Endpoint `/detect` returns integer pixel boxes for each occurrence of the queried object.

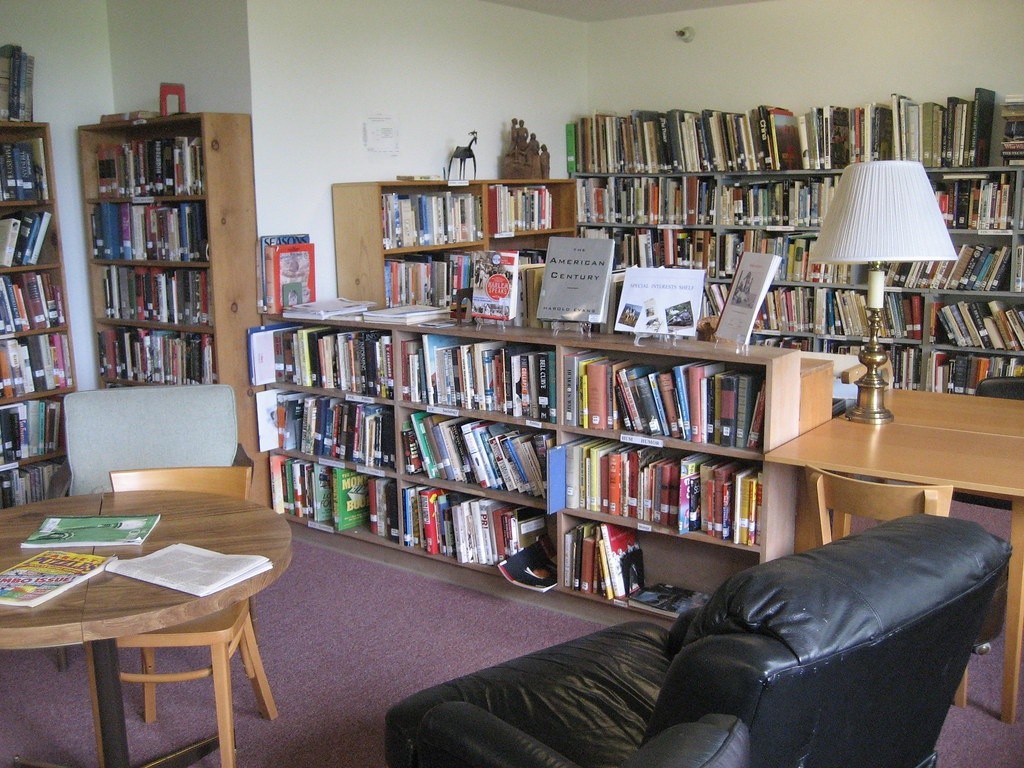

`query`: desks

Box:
[0,490,292,768]
[837,387,1024,437]
[765,417,1024,724]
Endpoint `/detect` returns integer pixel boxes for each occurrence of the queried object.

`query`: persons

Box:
[512,118,550,179]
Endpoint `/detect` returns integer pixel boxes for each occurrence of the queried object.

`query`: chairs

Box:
[952,377,1024,511]
[805,464,969,707]
[85,465,279,768]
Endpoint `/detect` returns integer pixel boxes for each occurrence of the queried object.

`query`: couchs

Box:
[385,515,1013,768]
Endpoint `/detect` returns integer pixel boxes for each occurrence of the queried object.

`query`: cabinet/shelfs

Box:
[0,122,77,473]
[332,179,577,307]
[77,113,271,509]
[799,358,834,435]
[571,166,1024,392]
[261,307,800,623]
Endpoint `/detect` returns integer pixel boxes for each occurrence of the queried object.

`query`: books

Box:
[0,210,52,268]
[563,87,1024,170]
[699,286,1024,400]
[0,550,119,607]
[579,225,1022,295]
[92,138,206,198]
[0,45,37,124]
[244,322,765,450]
[20,513,161,548]
[0,143,48,206]
[97,264,215,325]
[0,403,62,465]
[254,388,762,546]
[0,273,66,335]
[0,327,77,403]
[268,452,712,618]
[257,230,785,352]
[100,329,214,384]
[574,172,1012,234]
[92,202,210,263]
[0,461,69,509]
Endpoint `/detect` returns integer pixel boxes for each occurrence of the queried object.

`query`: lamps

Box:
[809,160,959,425]
